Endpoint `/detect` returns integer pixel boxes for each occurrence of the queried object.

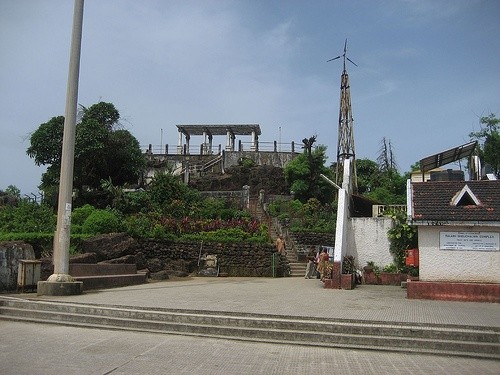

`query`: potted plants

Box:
[382,264,400,286]
[341,255,355,290]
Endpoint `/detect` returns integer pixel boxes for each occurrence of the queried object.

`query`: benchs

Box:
[69,263,146,291]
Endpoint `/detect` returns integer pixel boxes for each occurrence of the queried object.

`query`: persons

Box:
[276,234,286,254]
[304,246,329,279]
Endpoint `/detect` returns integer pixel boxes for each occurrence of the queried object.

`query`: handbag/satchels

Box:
[317,271,320,279]
